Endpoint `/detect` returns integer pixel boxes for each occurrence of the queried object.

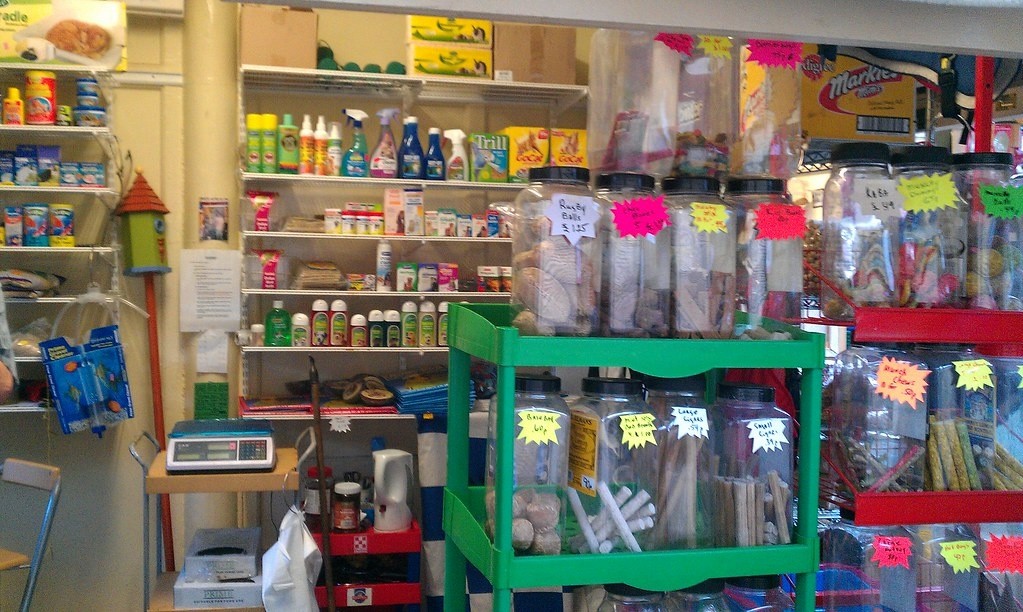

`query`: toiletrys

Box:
[250,299,448,347]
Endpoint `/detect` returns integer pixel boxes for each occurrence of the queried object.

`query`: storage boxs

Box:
[492,23,576,84]
[239,6,318,70]
[466,125,588,186]
[182,524,259,578]
[171,572,264,612]
[383,187,499,239]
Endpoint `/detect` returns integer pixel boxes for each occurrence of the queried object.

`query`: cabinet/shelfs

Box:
[803,307,1023,526]
[442,296,828,611]
[238,65,587,530]
[0,58,123,413]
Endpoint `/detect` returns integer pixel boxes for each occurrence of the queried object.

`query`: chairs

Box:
[0,459,62,612]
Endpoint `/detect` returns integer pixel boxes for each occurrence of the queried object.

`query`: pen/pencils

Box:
[363,478,371,490]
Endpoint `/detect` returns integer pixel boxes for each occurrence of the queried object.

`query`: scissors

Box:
[343,471,361,483]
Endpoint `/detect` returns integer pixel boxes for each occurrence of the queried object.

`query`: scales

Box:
[166,418,275,475]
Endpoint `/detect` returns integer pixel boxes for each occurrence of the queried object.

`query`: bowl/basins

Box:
[72,79,106,127]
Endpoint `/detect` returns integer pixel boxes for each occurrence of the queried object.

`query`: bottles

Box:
[247,113,277,174]
[251,324,264,347]
[265,301,291,347]
[587,28,802,177]
[818,342,1023,612]
[571,376,662,554]
[343,211,385,235]
[376,240,392,292]
[402,300,471,347]
[398,117,425,178]
[277,115,299,174]
[303,466,360,534]
[292,300,368,348]
[425,127,445,179]
[300,115,314,175]
[596,576,794,612]
[786,143,1023,315]
[643,373,792,549]
[485,373,572,556]
[315,115,330,175]
[511,167,805,343]
[3,70,56,125]
[328,123,343,176]
[368,310,402,347]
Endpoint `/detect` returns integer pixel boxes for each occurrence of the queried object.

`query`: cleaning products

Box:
[375,239,393,291]
[246,107,470,182]
[2,87,24,125]
